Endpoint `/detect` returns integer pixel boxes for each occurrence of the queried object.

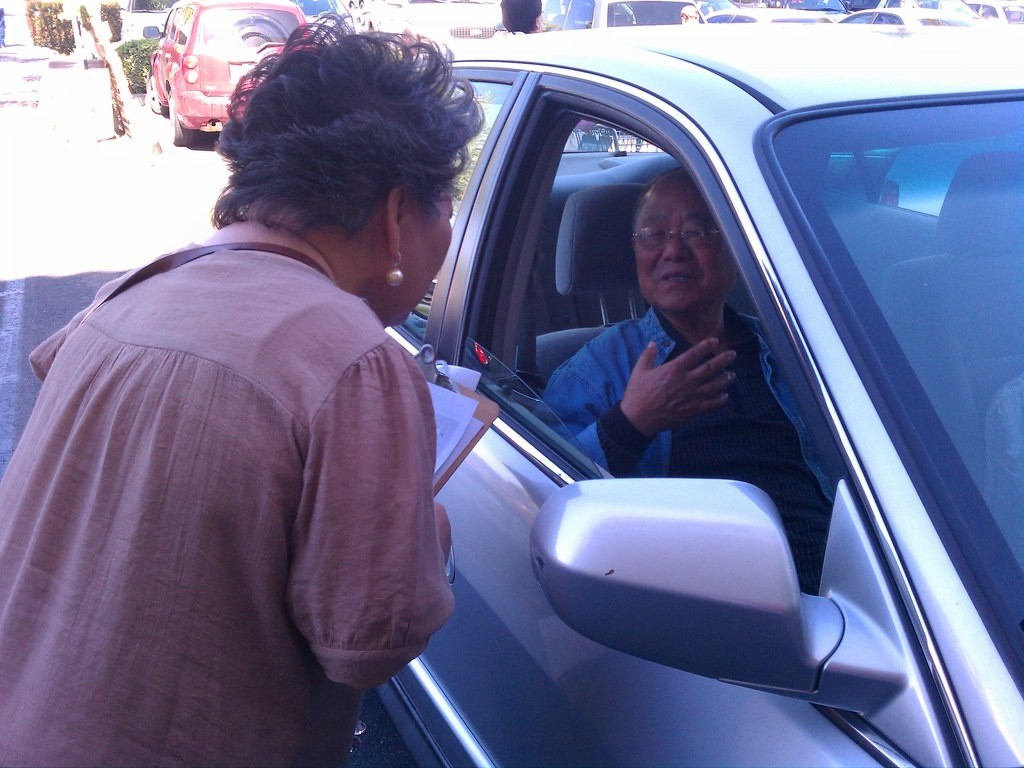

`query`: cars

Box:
[369,23,1023,768]
[143,0,322,148]
[291,0,1023,55]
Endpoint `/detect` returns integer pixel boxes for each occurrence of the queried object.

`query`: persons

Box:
[681,5,699,24]
[533,165,835,595]
[0,13,484,768]
[500,0,543,34]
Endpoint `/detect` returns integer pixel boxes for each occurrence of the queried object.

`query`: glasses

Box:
[631,225,720,252]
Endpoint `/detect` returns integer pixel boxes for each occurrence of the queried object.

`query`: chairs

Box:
[527,183,649,401]
[878,150,1024,398]
